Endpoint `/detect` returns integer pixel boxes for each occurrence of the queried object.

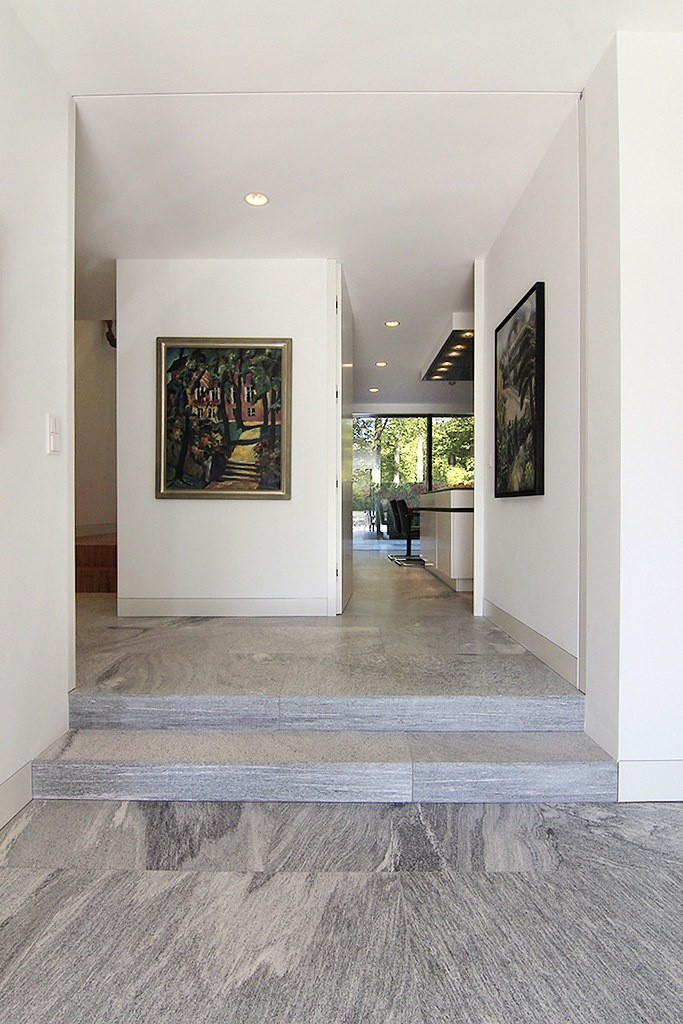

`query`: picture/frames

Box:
[494,281,544,498]
[156,336,292,500]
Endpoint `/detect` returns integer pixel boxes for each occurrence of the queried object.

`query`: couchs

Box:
[387,503,419,539]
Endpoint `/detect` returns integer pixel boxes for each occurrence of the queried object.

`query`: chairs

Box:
[374,502,387,537]
[387,500,419,561]
[395,499,425,567]
